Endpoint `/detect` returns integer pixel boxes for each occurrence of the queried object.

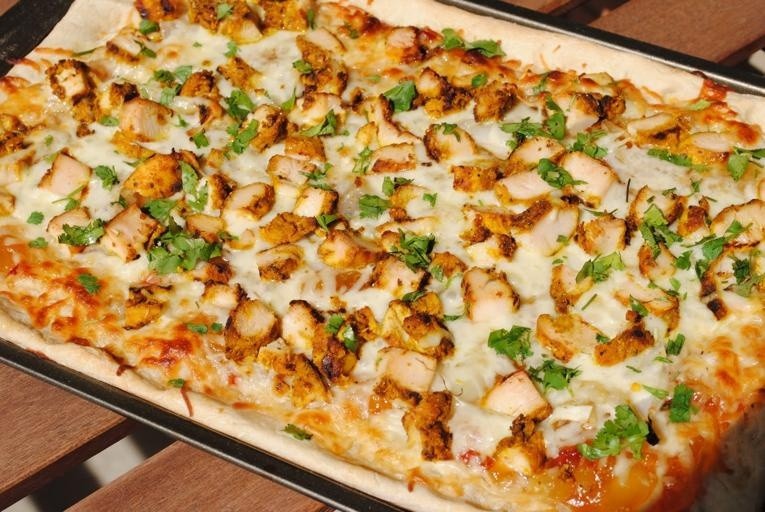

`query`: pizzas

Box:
[0,0,764,511]
